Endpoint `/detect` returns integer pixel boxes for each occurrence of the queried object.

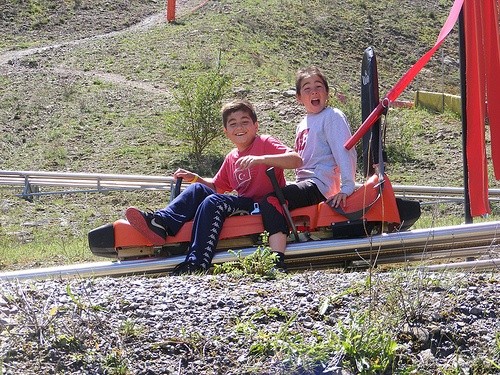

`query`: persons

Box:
[252,65,358,279]
[125,97,304,276]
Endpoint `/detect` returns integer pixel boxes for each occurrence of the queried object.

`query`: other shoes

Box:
[250,259,288,278]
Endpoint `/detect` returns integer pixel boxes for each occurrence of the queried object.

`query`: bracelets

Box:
[189,173,198,183]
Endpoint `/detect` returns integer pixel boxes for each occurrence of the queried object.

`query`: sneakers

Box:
[126,206,166,245]
[172,261,204,276]
